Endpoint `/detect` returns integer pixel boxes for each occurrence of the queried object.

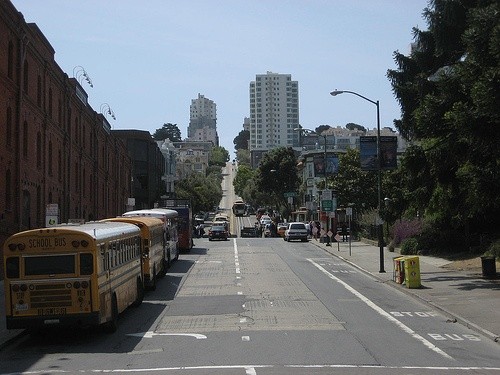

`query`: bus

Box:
[3,208,178,333]
[232,200,255,216]
[164,207,195,253]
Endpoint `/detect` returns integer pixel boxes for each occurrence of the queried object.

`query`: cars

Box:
[337,228,351,235]
[194,207,232,241]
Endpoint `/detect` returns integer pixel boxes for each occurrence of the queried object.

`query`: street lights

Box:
[271,169,292,209]
[330,88,387,273]
[294,127,331,246]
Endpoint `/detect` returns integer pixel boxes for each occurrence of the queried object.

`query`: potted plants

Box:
[387,241,396,252]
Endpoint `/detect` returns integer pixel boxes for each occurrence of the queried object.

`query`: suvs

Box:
[241,225,260,237]
[256,208,309,242]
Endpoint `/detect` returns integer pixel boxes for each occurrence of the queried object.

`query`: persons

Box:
[312,225,319,241]
[196,226,199,232]
[305,221,313,236]
[270,221,275,238]
[199,225,203,237]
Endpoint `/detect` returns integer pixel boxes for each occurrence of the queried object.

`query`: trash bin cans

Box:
[482,256,495,277]
